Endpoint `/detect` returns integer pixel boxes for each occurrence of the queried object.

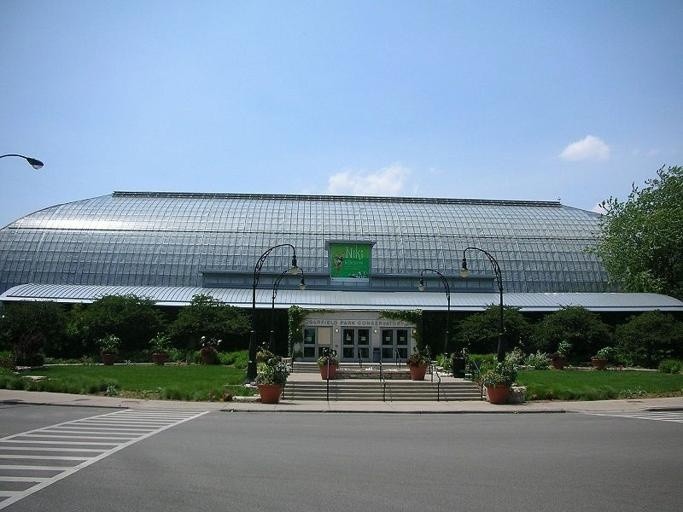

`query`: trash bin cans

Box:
[453,357,465,378]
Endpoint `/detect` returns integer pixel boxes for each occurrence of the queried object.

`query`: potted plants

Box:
[254,357,288,403]
[591,346,612,369]
[484,355,515,403]
[406,345,431,379]
[148,332,171,364]
[510,382,527,403]
[318,348,339,380]
[98,334,120,366]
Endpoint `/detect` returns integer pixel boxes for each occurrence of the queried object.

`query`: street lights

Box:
[419,269,449,358]
[0,154,44,169]
[272,267,304,309]
[460,247,505,361]
[245,244,298,384]
